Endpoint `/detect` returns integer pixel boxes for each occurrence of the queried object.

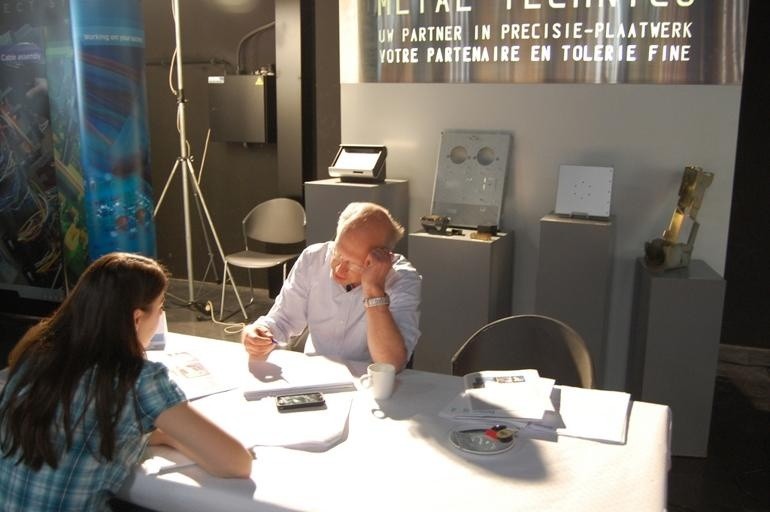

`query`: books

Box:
[140,308,357,477]
[438,366,633,444]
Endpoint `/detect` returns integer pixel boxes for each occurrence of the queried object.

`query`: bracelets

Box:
[361,291,390,314]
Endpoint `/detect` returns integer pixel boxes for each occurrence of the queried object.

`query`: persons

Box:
[240,200,426,375]
[2,252,253,509]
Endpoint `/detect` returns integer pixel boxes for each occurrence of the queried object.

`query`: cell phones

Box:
[276,392,325,410]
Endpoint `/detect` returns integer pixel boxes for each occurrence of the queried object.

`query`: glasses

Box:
[326,239,364,275]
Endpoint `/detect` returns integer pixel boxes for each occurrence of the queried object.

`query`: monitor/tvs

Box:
[327,143,387,184]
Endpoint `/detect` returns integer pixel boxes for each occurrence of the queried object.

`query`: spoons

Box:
[459,424,507,434]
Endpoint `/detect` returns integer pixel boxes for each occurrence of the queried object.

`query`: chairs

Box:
[216,196,306,323]
[447,312,596,389]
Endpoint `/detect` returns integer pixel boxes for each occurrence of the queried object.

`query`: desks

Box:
[0,332,675,512]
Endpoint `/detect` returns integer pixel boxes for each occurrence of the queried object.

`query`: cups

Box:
[359,362,395,399]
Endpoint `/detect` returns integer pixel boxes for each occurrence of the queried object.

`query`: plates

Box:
[447,422,516,455]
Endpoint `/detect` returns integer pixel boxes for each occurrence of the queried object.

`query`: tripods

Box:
[152,0,248,320]
